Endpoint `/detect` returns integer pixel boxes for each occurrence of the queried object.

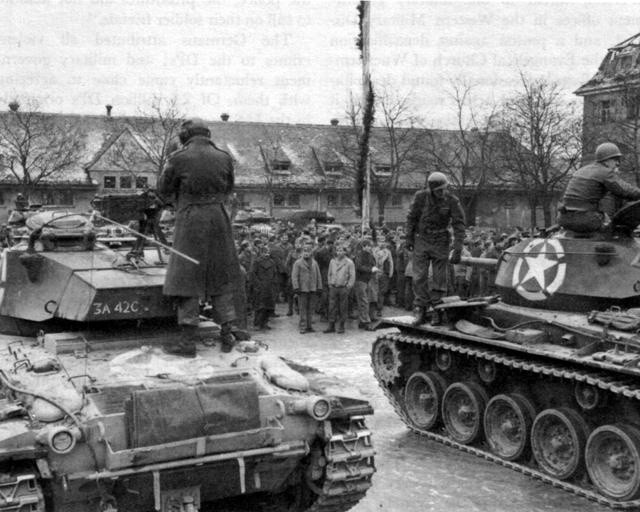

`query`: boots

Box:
[431,310,440,325]
[413,307,425,326]
[287,299,293,315]
[337,321,344,333]
[323,323,335,333]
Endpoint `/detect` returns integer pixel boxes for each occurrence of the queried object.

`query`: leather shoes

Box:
[270,311,280,318]
[223,334,235,352]
[299,328,305,333]
[307,326,315,332]
[258,324,269,330]
[163,341,196,358]
[359,322,364,327]
[365,324,375,330]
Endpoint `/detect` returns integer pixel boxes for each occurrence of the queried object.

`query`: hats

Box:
[180,118,211,144]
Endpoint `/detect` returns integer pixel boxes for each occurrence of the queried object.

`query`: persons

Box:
[158,221,173,245]
[405,171,465,327]
[557,142,640,241]
[231,222,434,334]
[449,226,536,299]
[156,117,241,358]
[1,191,29,249]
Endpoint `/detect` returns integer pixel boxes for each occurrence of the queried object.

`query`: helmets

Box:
[596,143,622,162]
[428,172,449,191]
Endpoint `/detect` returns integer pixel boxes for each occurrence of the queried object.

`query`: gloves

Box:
[450,249,461,263]
[406,240,413,251]
